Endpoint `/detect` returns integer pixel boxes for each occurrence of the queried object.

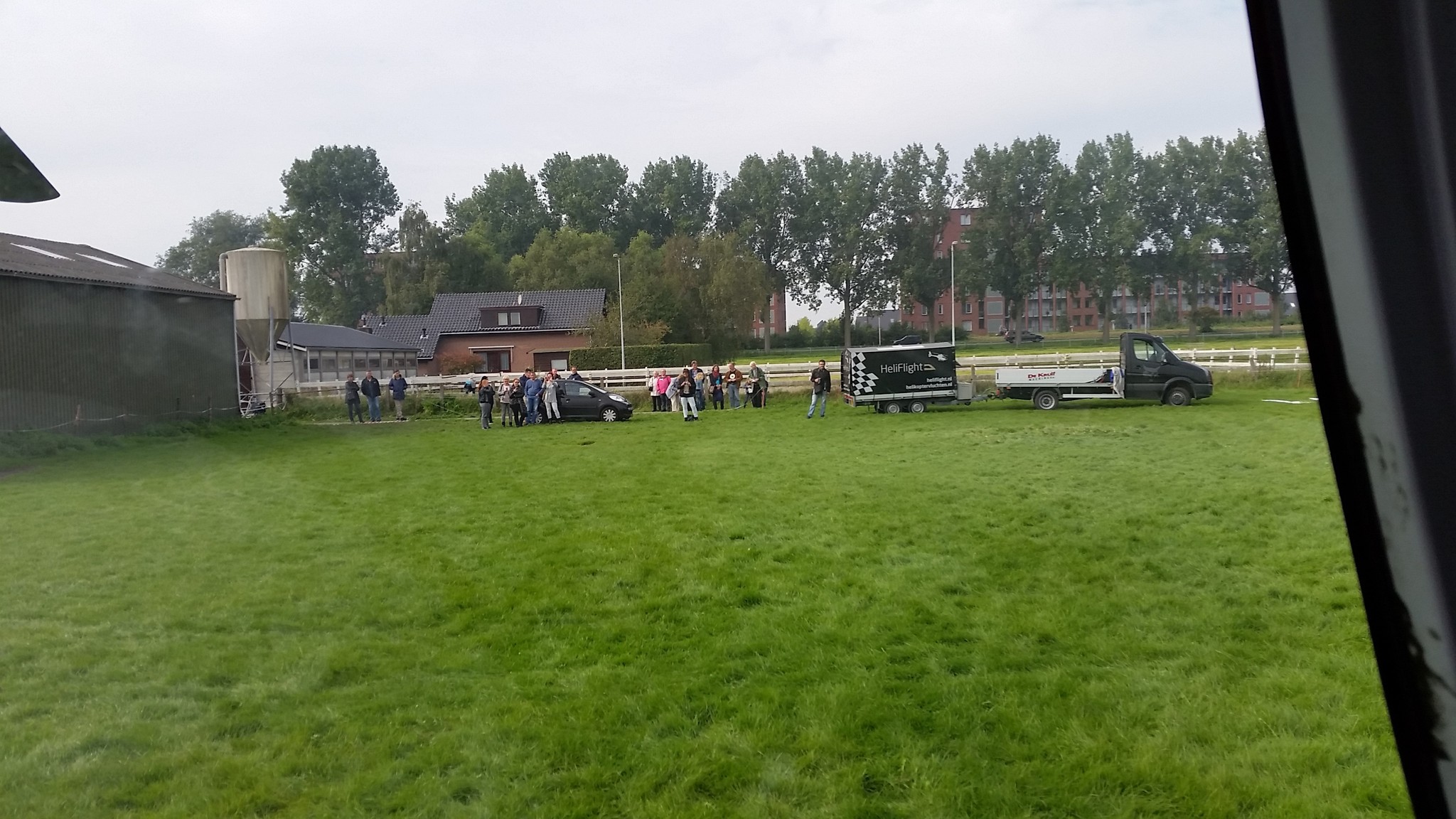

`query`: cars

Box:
[525,379,633,424]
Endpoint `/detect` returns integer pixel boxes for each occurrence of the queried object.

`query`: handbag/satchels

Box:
[542,391,546,401]
[708,386,715,394]
[814,384,822,395]
[649,387,653,391]
[347,391,355,400]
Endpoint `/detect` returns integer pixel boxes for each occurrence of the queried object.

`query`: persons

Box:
[805,359,831,420]
[541,372,565,425]
[360,370,382,422]
[477,375,496,430]
[524,371,543,426]
[549,368,562,380]
[508,378,528,427]
[497,376,514,427]
[568,366,583,381]
[999,325,1008,336]
[345,374,365,423]
[388,369,408,422]
[647,360,768,412]
[519,368,532,389]
[677,368,700,423]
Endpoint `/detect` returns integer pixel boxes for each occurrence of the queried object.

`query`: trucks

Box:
[995,332,1213,411]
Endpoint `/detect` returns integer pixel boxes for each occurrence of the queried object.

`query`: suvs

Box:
[893,335,922,346]
[1004,330,1044,343]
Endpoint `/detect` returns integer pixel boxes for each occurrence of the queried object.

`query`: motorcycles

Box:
[995,329,1008,336]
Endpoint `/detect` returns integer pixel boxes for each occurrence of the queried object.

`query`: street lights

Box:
[613,253,627,387]
[951,241,958,345]
[878,315,883,345]
